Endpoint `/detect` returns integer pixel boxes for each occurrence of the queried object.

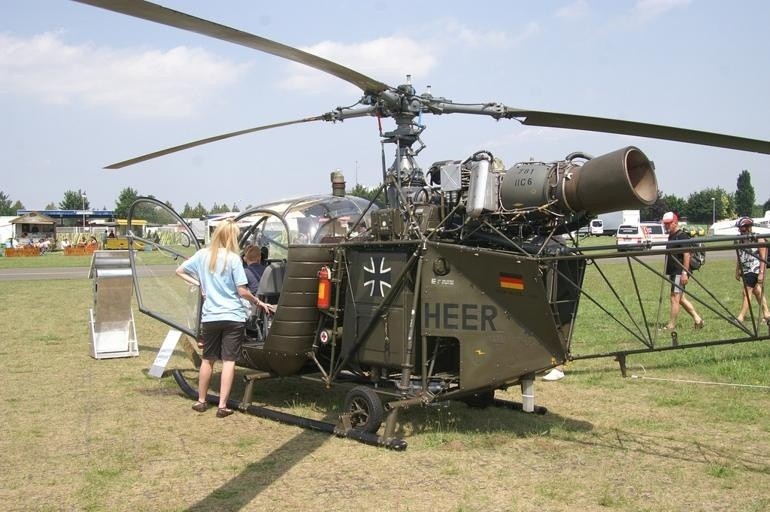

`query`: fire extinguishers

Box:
[316,265,332,310]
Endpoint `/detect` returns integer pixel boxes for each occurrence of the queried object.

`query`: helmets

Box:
[735,216,753,227]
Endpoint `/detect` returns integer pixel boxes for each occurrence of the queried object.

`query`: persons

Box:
[241,244,267,316]
[175,218,275,418]
[108,230,114,238]
[99,230,107,249]
[8,232,97,256]
[731,217,770,326]
[658,211,706,334]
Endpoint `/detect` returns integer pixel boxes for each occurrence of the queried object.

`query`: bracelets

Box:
[681,270,689,275]
[760,270,765,272]
[256,298,260,305]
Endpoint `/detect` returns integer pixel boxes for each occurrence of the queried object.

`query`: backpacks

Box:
[751,233,770,268]
[676,231,705,270]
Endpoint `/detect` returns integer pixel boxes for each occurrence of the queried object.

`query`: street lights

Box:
[81,191,87,227]
[711,197,715,224]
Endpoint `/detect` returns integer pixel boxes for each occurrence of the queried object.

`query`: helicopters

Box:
[70,0,770,451]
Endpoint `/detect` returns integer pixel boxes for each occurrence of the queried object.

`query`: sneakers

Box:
[658,325,676,332]
[732,319,744,325]
[759,316,770,325]
[192,401,208,411]
[542,369,565,381]
[216,407,234,418]
[690,320,705,334]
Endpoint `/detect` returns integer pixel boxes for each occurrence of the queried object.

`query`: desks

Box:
[106,237,144,250]
[63,246,95,256]
[4,247,39,256]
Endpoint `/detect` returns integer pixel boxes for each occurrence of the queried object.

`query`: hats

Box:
[660,211,678,224]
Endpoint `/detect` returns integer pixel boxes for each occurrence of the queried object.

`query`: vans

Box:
[578,218,604,237]
[615,222,688,250]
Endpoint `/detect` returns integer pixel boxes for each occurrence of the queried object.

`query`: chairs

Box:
[243,264,284,341]
[40,245,48,255]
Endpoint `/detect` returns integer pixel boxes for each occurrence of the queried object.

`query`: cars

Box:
[684,225,705,237]
